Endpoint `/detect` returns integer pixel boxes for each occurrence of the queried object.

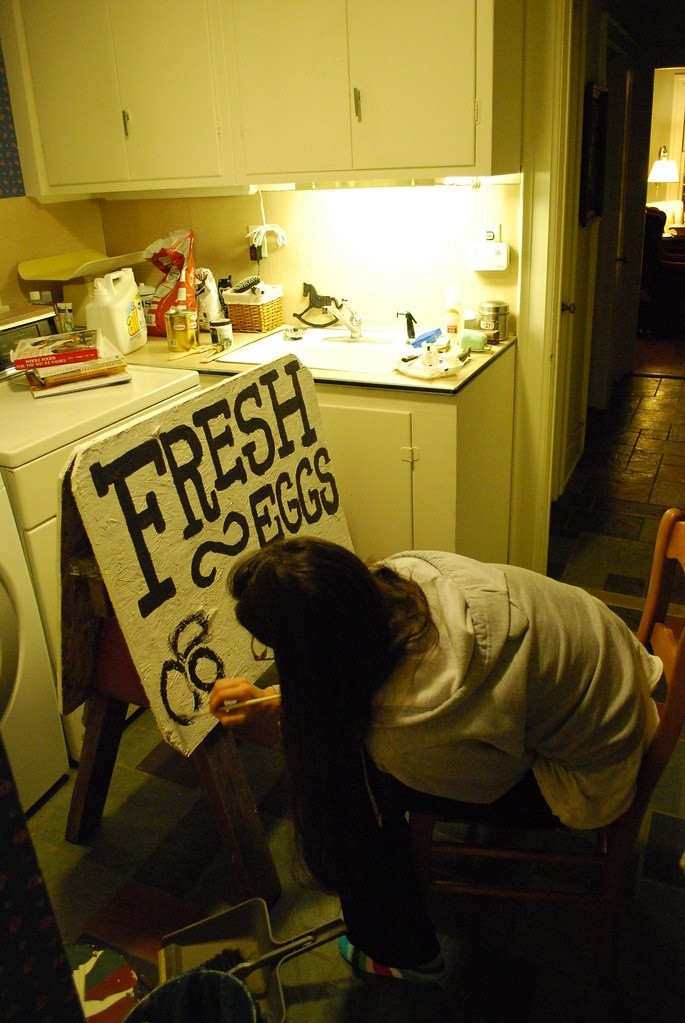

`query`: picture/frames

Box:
[580,79,609,226]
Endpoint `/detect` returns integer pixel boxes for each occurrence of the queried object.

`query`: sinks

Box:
[238,341,394,371]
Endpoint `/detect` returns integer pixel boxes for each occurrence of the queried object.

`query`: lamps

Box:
[648,145,679,202]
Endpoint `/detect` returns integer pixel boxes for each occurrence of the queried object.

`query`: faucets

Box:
[321,296,363,340]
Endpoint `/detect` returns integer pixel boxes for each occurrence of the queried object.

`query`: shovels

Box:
[156,898,346,1023]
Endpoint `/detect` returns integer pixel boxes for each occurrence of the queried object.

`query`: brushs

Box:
[233,276,260,293]
[167,344,211,361]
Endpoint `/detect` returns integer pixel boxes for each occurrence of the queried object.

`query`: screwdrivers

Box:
[202,337,232,359]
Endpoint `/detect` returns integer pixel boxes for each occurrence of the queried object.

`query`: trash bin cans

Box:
[120,970,263,1023]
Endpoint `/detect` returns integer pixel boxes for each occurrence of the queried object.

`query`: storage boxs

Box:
[12,327,104,368]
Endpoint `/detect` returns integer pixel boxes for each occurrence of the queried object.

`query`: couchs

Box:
[639,206,685,341]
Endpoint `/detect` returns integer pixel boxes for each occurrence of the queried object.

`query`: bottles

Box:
[84,267,148,356]
[30,290,74,337]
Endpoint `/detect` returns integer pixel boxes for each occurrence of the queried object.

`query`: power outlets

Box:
[248,225,268,258]
[477,223,503,244]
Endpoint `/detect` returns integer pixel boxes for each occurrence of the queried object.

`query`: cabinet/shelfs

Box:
[0,0,529,204]
[194,344,520,564]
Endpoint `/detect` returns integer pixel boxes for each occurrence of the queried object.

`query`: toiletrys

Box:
[446,308,461,345]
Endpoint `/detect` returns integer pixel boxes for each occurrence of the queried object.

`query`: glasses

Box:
[251,635,275,661]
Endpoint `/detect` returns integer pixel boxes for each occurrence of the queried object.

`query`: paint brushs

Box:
[180,695,280,722]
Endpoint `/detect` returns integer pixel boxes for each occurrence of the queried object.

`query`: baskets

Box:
[222,285,285,333]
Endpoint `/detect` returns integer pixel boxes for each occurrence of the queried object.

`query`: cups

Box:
[477,300,510,342]
[218,279,231,318]
[137,282,156,323]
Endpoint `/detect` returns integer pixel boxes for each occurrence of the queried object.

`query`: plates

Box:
[470,344,491,351]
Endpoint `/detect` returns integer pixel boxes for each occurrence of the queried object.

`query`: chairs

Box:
[406,504,685,993]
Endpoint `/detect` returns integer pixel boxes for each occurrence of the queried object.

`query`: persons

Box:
[208,537,655,997]
[31,333,71,349]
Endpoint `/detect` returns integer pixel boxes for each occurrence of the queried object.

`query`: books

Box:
[13,328,133,400]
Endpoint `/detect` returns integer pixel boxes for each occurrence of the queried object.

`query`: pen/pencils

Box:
[401,350,426,362]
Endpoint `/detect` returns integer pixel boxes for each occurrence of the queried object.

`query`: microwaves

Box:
[0,306,62,383]
